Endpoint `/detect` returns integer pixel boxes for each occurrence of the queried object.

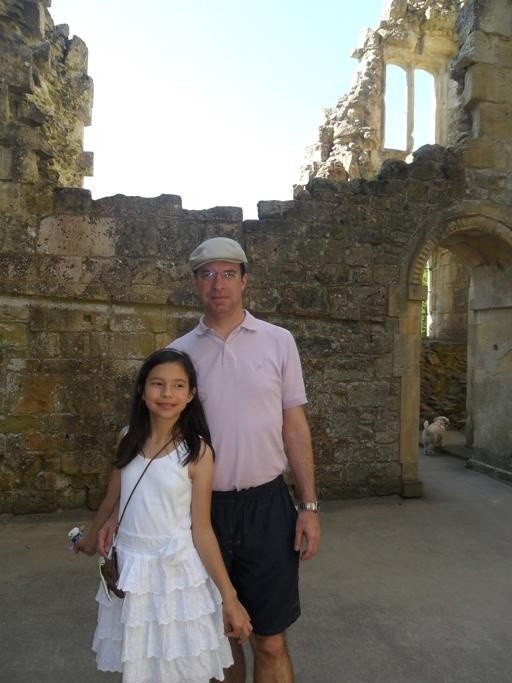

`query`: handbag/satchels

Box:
[99,544,127,599]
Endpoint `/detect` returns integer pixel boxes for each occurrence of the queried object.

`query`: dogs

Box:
[421,415,450,455]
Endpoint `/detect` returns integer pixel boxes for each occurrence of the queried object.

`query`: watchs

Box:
[299,501,321,513]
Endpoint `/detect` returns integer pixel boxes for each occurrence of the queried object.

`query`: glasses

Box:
[197,270,239,280]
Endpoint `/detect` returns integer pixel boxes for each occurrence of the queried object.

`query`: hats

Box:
[185,235,250,273]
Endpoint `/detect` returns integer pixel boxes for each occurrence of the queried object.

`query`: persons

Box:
[98,238,321,683]
[74,347,254,683]
